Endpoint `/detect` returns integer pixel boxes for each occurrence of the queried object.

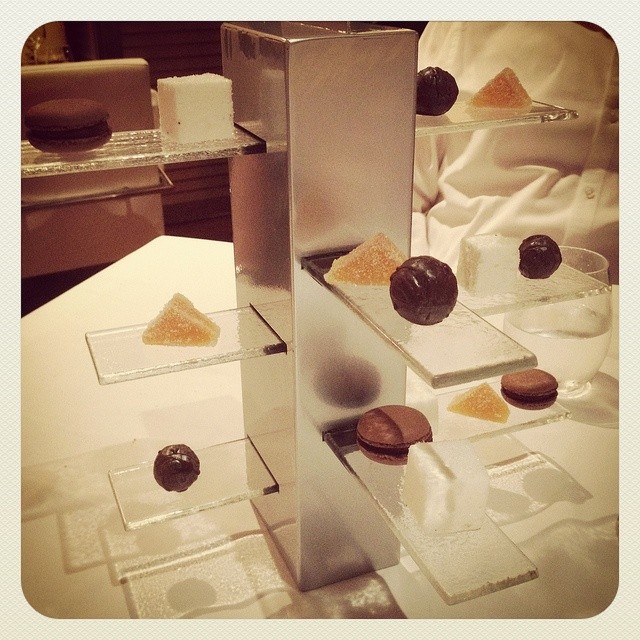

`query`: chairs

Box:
[20,57,168,281]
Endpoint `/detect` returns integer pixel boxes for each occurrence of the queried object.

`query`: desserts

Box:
[501,368,559,410]
[142,292,221,347]
[153,443,200,493]
[25,99,113,154]
[390,256,459,326]
[323,232,407,287]
[415,65,459,116]
[456,232,521,298]
[357,405,433,465]
[157,72,236,145]
[518,234,562,279]
[468,66,532,109]
[403,442,490,535]
[446,382,510,423]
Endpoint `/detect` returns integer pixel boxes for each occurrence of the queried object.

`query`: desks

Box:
[17,231,618,623]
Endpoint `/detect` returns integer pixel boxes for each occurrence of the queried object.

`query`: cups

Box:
[503,247,612,399]
